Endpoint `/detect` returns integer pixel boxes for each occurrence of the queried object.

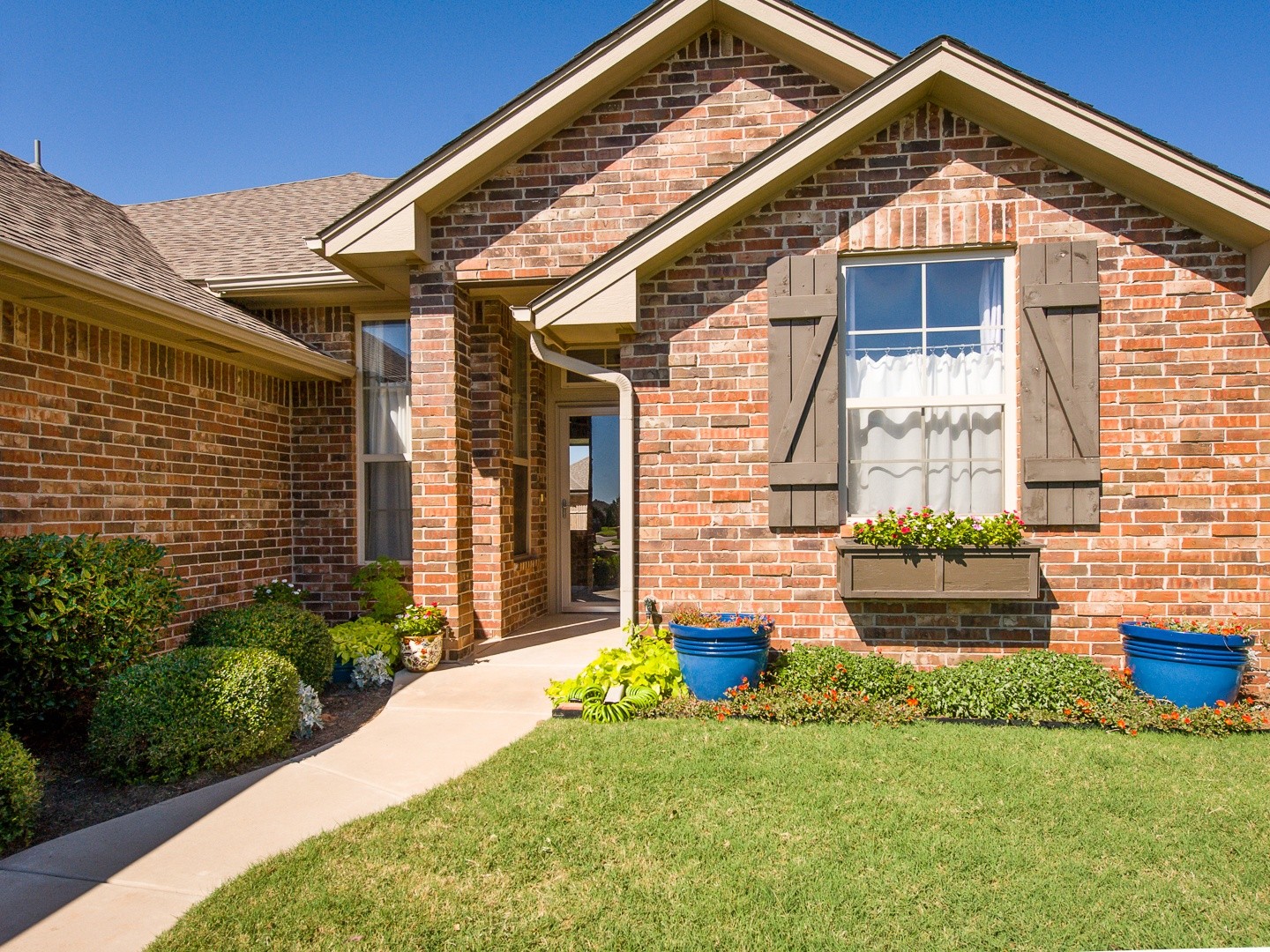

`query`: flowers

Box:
[394,600,447,637]
[851,504,1026,552]
[672,600,775,638]
[1135,611,1259,641]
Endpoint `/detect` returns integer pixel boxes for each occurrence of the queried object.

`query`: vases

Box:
[396,631,444,672]
[832,537,1047,599]
[1118,621,1256,711]
[669,612,773,701]
